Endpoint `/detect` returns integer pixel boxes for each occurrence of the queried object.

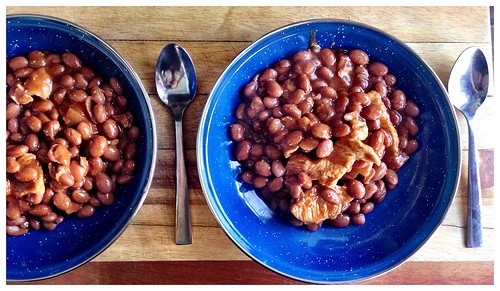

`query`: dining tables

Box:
[5,6,492,284]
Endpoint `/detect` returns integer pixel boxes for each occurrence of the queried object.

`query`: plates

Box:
[197,15,463,285]
[6,12,160,283]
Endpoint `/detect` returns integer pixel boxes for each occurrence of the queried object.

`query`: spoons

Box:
[448,44,491,250]
[154,42,196,246]
